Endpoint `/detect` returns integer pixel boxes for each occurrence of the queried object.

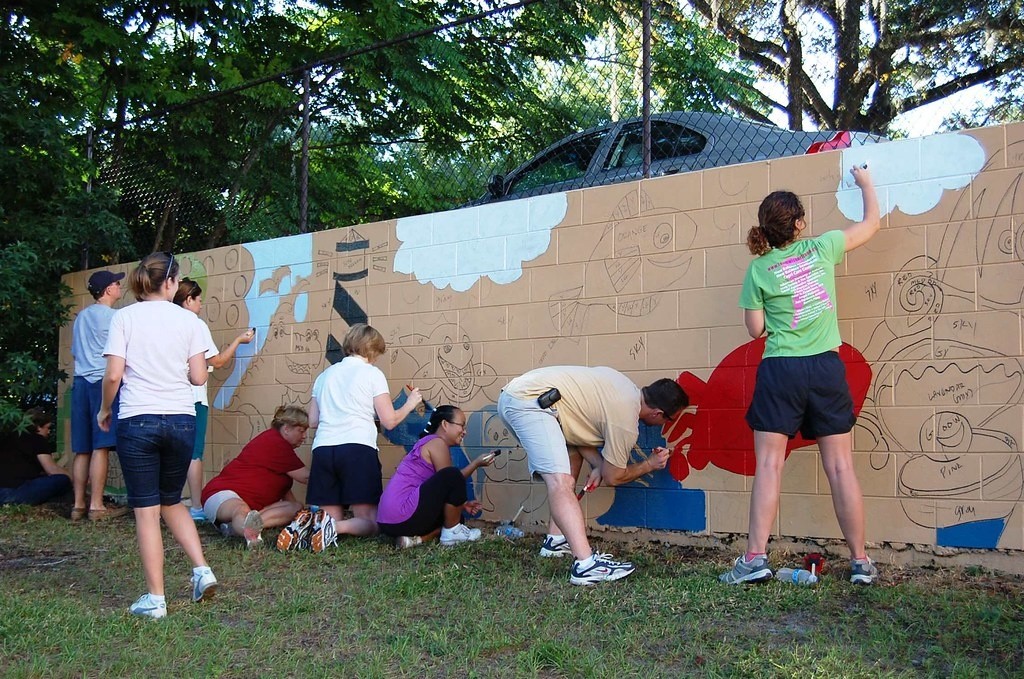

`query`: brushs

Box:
[577,485,588,500]
[482,449,502,461]
[862,164,868,169]
[247,328,254,335]
[651,448,674,454]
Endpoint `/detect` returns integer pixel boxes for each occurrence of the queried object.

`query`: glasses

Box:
[164,251,174,280]
[450,422,466,430]
[188,283,199,295]
[665,413,674,422]
[111,281,120,286]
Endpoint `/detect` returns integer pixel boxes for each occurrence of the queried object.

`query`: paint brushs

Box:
[406,384,425,403]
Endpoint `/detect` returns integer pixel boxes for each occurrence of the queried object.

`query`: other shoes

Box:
[71,507,87,522]
[87,503,127,521]
[190,507,206,521]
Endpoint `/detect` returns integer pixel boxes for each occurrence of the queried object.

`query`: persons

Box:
[0,271,254,521]
[200,406,312,552]
[498,366,691,585]
[97,251,217,619]
[277,323,422,553]
[376,405,496,550]
[718,164,880,585]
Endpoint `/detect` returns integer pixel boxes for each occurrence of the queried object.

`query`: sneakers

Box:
[397,536,422,549]
[308,509,337,555]
[244,510,264,550]
[849,551,877,584]
[440,523,481,545]
[718,554,773,586]
[569,552,636,586]
[131,594,168,619]
[220,523,234,537]
[276,508,313,554]
[540,534,572,558]
[191,569,217,603]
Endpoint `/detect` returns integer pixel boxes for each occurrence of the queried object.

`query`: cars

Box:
[454,111,888,210]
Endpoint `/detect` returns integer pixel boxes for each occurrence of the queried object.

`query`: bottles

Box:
[776,567,818,585]
[495,525,524,540]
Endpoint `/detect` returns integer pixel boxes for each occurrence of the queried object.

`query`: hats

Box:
[88,271,125,296]
